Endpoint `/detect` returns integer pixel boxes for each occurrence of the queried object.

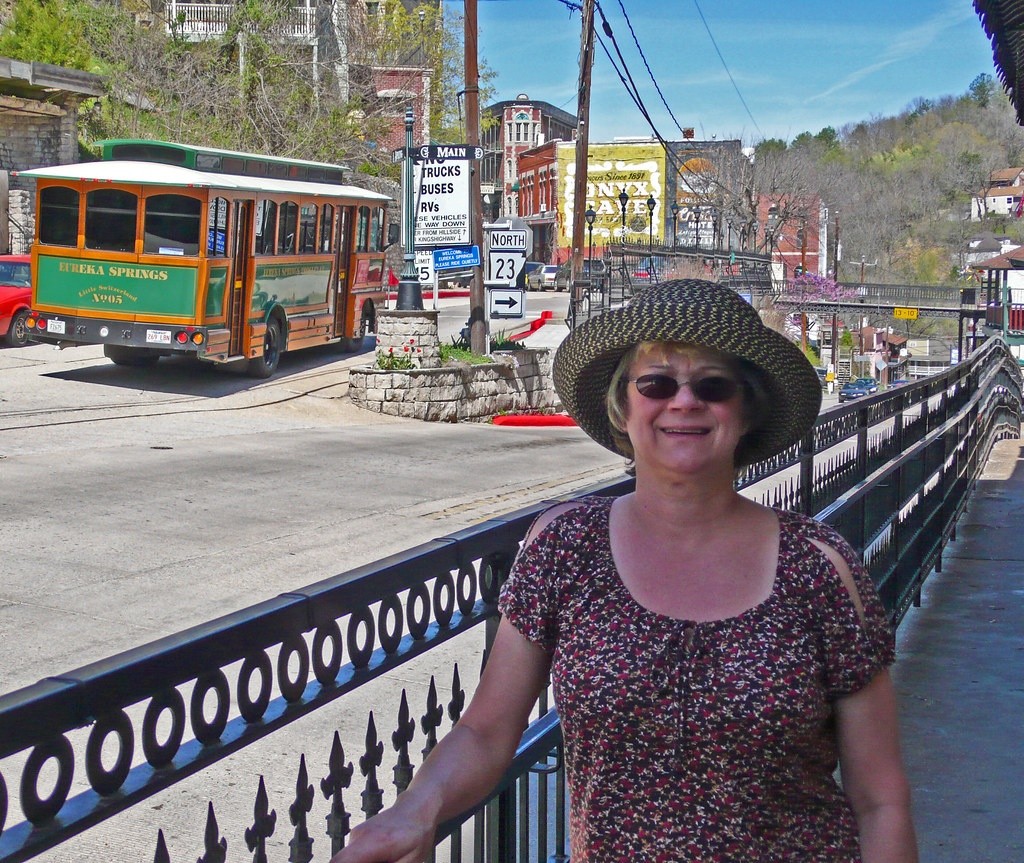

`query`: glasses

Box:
[616,370,748,405]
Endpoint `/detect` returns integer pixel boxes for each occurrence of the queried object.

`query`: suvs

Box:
[555,258,608,293]
[815,368,834,393]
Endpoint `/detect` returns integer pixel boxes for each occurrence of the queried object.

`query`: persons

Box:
[868,380,873,384]
[857,385,862,389]
[848,375,856,383]
[327,279,920,863]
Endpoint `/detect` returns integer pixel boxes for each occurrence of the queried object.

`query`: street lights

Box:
[585,205,596,321]
[693,204,700,279]
[710,208,718,277]
[671,201,679,280]
[646,194,660,286]
[739,217,747,276]
[618,188,636,308]
[726,212,735,277]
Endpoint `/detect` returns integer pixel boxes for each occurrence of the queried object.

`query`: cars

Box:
[523,262,546,291]
[838,382,868,404]
[528,265,561,292]
[888,380,911,390]
[793,267,808,279]
[0,253,33,347]
[853,378,880,395]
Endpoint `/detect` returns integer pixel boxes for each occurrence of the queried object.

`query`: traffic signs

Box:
[484,229,528,319]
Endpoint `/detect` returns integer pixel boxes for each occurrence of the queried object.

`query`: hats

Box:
[552,276,823,470]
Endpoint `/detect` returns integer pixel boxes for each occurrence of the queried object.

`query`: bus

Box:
[7,138,400,378]
